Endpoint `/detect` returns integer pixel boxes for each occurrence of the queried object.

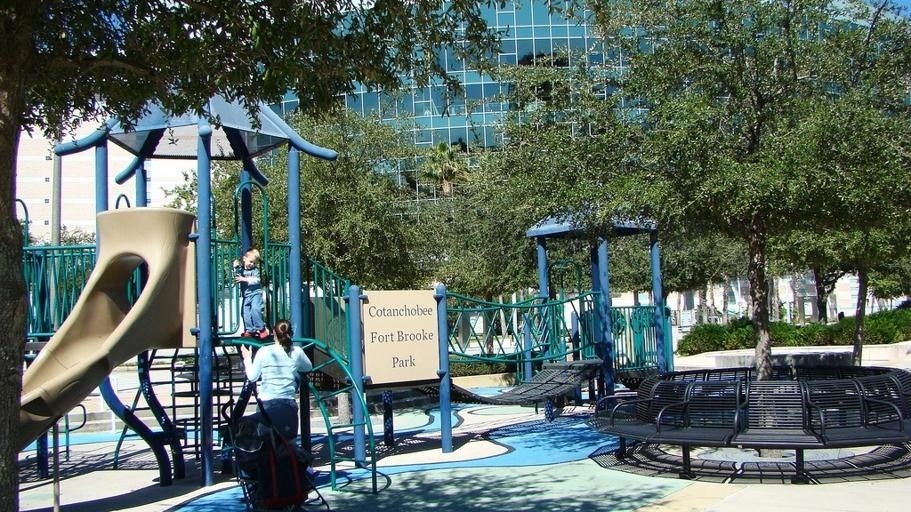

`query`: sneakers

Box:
[241,330,255,337]
[260,329,268,339]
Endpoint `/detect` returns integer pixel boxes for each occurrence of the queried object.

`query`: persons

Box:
[233,248,270,339]
[241,320,313,442]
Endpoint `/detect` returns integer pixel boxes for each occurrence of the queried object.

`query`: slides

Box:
[16,208,196,455]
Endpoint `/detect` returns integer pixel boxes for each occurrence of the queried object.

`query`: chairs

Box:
[593,365,911,486]
